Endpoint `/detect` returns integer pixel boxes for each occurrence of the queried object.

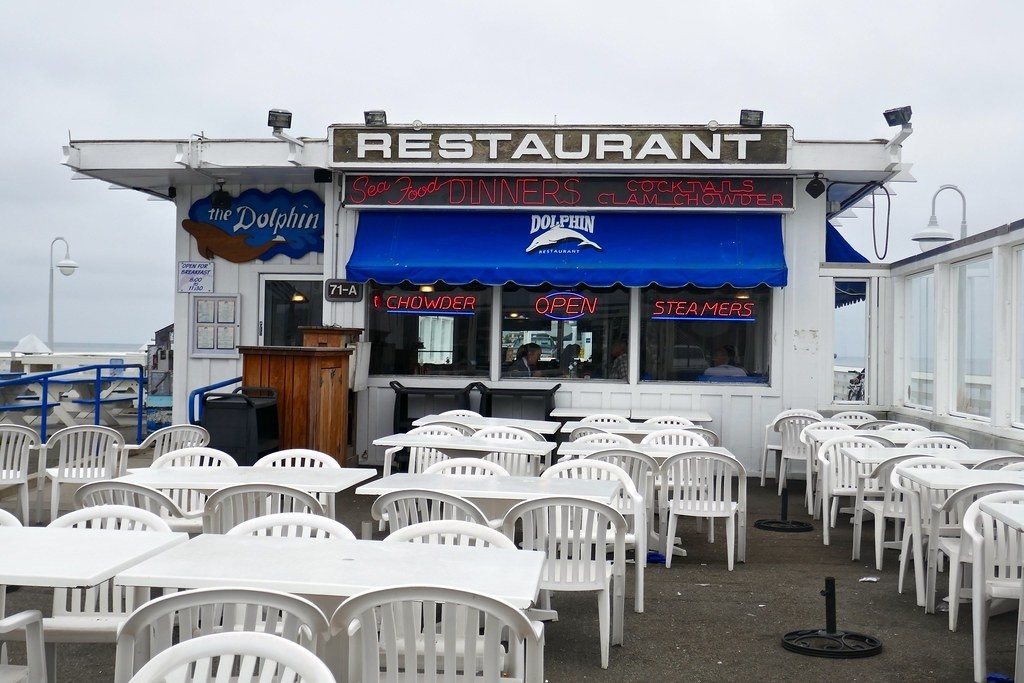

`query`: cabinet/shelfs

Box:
[389,380,561,475]
[201,324,366,504]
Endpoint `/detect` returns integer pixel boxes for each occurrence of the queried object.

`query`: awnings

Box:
[345,210,878,309]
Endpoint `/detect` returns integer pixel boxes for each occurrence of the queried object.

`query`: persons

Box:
[505,339,747,377]
[853,368,865,384]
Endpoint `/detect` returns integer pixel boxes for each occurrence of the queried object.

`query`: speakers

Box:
[314,169,333,183]
[806,179,826,198]
[211,191,232,209]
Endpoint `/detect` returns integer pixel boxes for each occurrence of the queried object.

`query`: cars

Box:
[511,337,557,360]
[665,344,711,371]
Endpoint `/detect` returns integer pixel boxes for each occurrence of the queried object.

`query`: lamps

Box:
[741,109,763,127]
[883,106,912,127]
[268,108,292,128]
[364,111,388,126]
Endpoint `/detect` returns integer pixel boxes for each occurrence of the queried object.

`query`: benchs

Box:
[354,473,622,644]
[71,396,138,404]
[14,395,69,400]
[0,403,60,410]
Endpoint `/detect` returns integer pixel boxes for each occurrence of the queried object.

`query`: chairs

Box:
[0,410,746,683]
[761,409,1024,683]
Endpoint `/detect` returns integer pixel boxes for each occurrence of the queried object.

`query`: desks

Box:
[0,526,189,667]
[372,433,557,474]
[557,442,735,557]
[0,372,148,456]
[786,418,901,428]
[411,415,562,437]
[804,429,967,523]
[896,468,1024,604]
[840,448,1024,560]
[115,533,547,683]
[549,407,712,422]
[108,467,380,627]
[560,421,703,514]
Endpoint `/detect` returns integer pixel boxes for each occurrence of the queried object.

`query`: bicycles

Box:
[847,370,865,401]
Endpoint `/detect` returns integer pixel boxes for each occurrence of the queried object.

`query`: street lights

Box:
[48,237,79,354]
[911,185,968,412]
